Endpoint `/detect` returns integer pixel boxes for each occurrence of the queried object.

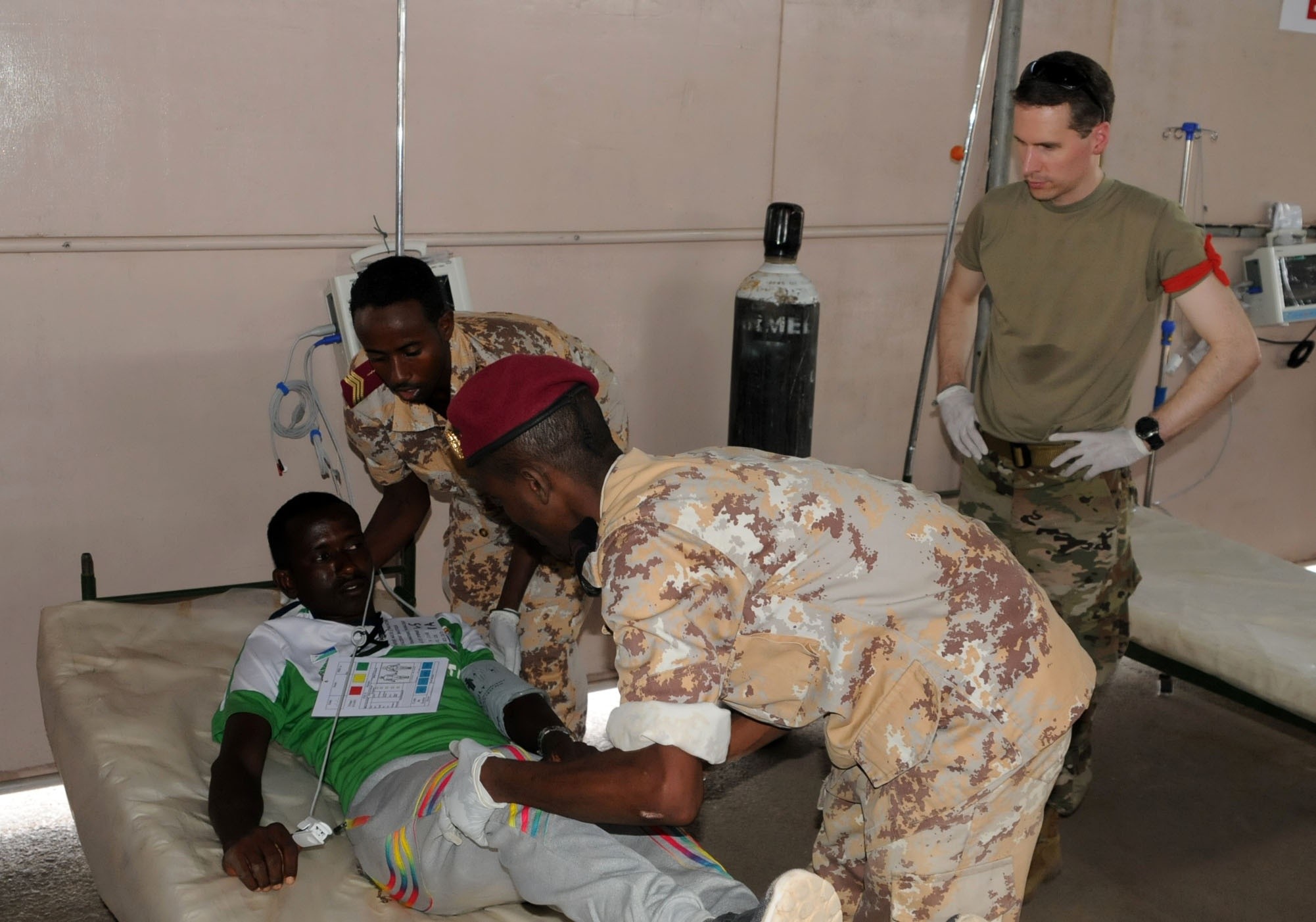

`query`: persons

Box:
[448,352,1096,921]
[209,492,844,921]
[340,256,629,743]
[937,50,1261,905]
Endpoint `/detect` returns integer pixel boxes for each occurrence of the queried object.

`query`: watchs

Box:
[1135,416,1165,451]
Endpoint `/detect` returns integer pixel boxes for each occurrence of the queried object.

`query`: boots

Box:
[1024,806,1063,897]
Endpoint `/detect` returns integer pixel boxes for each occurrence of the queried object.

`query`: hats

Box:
[447,354,599,469]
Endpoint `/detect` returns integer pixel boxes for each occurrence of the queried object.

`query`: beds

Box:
[36,541,572,922]
[1125,503,1316,740]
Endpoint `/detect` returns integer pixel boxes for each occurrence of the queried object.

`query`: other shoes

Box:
[734,867,843,921]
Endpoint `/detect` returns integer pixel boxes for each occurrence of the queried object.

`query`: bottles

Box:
[729,201,821,457]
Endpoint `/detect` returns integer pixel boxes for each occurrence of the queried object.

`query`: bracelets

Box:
[536,725,577,757]
